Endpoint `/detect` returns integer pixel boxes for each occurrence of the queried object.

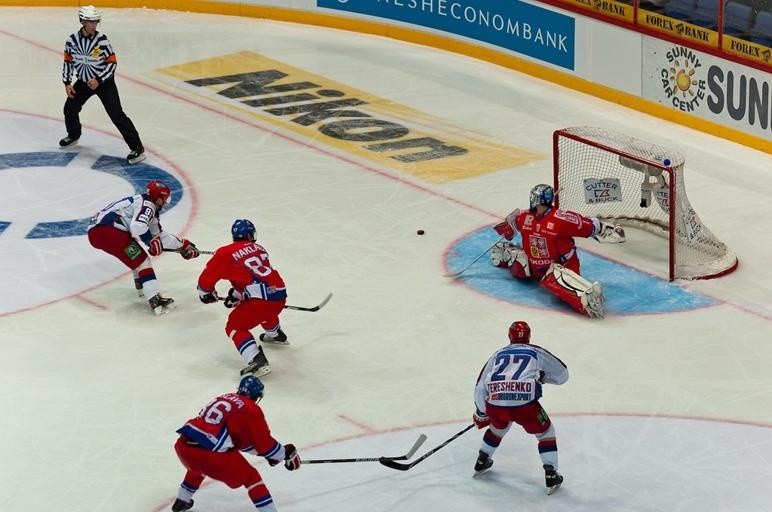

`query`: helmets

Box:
[236,375,265,402]
[231,219,255,242]
[529,183,554,209]
[147,181,170,210]
[508,321,531,343]
[78,5,101,23]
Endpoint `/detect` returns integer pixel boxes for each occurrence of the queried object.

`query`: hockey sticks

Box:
[442,186,564,279]
[218,292,333,313]
[379,423,476,471]
[300,434,427,465]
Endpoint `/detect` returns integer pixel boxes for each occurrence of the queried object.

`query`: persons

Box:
[198,219,290,378]
[89,180,200,316]
[58,5,146,163]
[172,375,301,512]
[473,321,569,497]
[492,184,626,319]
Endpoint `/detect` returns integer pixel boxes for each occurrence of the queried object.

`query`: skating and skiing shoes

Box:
[545,469,564,494]
[127,146,146,164]
[240,347,272,379]
[172,499,194,512]
[260,328,288,344]
[473,457,493,479]
[59,136,80,148]
[148,295,174,315]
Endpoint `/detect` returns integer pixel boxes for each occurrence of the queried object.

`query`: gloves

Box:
[225,287,242,308]
[179,239,199,259]
[198,287,219,303]
[280,443,301,471]
[149,236,163,256]
[600,222,625,243]
[473,411,490,428]
